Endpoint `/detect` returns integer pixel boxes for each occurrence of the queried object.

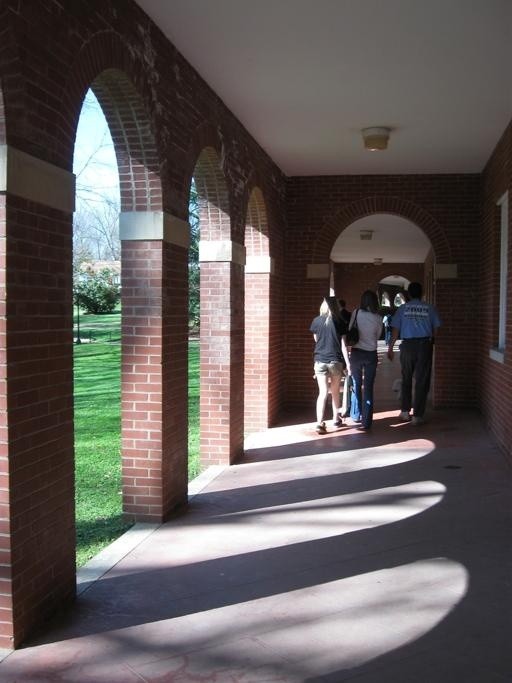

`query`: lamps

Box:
[362,124,391,152]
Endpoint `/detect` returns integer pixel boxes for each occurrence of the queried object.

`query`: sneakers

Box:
[410,416,426,427]
[398,414,410,421]
[317,422,327,434]
[335,420,344,429]
[354,418,369,432]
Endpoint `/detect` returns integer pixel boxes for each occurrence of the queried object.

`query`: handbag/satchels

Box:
[345,308,360,346]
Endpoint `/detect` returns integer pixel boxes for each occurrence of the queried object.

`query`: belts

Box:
[402,337,434,345]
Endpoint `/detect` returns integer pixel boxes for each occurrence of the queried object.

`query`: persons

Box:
[387,278,442,427]
[382,309,392,345]
[308,295,352,435]
[343,288,384,430]
[337,299,352,325]
